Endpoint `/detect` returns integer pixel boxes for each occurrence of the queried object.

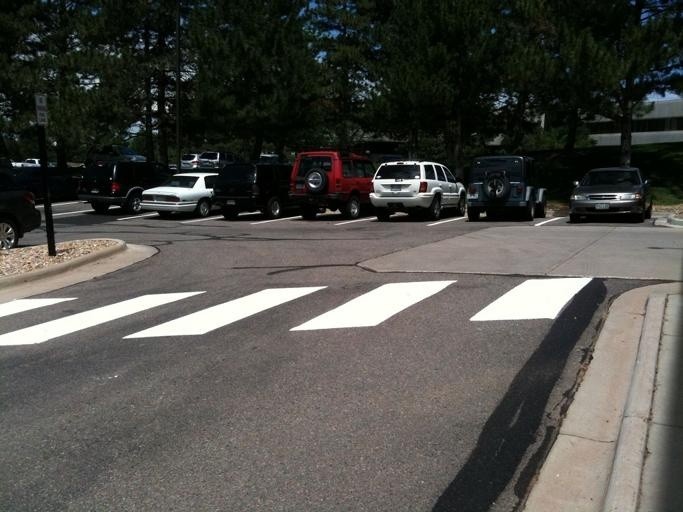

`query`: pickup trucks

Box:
[12,158,40,168]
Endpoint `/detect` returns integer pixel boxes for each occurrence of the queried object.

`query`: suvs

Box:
[79,161,164,215]
[466,156,547,222]
[170,152,234,171]
[212,163,292,220]
[290,151,376,220]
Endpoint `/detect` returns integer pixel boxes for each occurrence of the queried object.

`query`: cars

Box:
[570,168,653,224]
[369,161,467,221]
[0,188,42,249]
[141,172,218,217]
